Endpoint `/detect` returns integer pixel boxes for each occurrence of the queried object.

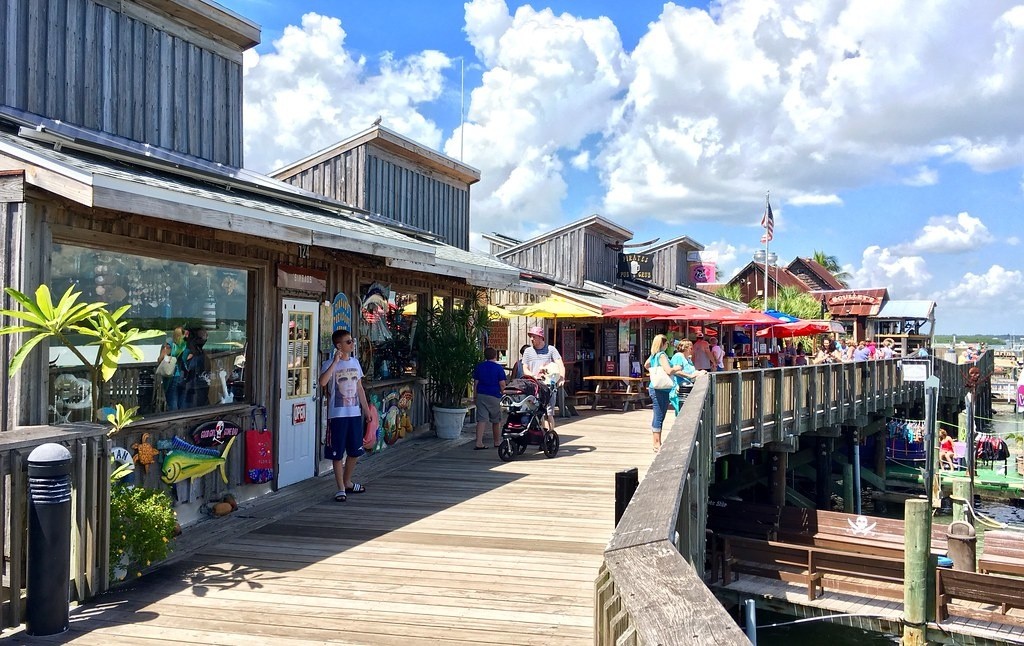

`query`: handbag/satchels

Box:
[155,342,177,378]
[244,406,274,484]
[648,352,674,389]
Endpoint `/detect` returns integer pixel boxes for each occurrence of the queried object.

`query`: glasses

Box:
[865,341,870,342]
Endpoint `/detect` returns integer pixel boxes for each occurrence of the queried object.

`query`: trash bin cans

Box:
[946,520,977,572]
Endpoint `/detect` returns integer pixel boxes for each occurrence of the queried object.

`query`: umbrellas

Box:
[604,302,829,370]
[401,296,512,322]
[509,297,603,346]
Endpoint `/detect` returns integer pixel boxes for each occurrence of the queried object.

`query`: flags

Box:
[761,203,774,243]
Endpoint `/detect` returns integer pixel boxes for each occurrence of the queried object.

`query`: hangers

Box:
[889,417,924,427]
[976,431,1002,441]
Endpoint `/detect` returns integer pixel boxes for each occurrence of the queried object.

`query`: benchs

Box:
[460,398,475,424]
[706,529,722,583]
[935,566,1024,626]
[978,530,1024,577]
[777,506,949,560]
[575,388,650,396]
[717,533,906,607]
[711,497,779,540]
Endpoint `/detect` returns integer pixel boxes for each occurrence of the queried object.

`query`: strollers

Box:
[496,375,560,461]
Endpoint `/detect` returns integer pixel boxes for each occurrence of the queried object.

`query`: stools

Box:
[566,395,588,405]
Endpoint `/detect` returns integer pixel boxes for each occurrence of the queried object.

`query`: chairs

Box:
[945,441,967,471]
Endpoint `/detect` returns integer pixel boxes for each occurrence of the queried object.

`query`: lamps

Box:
[757,290,763,296]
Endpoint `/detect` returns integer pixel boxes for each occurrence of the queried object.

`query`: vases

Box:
[113,549,129,583]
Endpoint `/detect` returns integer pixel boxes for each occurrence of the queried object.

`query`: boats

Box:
[828,429,1024,492]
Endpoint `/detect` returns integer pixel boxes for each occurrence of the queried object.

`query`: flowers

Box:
[0,282,177,586]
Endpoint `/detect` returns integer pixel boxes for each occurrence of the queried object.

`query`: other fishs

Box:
[160,436,236,485]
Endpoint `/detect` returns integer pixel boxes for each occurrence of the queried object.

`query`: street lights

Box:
[753,248,779,312]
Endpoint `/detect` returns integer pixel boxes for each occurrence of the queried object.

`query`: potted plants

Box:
[403,299,495,441]
[1004,434,1024,476]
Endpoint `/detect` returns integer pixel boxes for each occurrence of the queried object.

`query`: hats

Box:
[528,327,545,341]
[696,333,703,337]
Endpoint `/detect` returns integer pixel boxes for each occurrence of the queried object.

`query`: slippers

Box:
[474,445,499,450]
[345,484,365,493]
[334,491,346,502]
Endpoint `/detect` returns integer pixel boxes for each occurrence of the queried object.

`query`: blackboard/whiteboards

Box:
[561,328,576,364]
[604,328,618,356]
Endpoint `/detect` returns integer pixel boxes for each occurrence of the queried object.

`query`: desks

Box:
[585,375,646,411]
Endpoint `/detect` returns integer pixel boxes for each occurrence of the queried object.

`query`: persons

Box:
[522,327,565,430]
[320,329,372,502]
[159,326,211,411]
[958,342,987,364]
[473,347,507,450]
[939,428,955,471]
[644,334,681,452]
[511,344,531,379]
[671,331,902,412]
